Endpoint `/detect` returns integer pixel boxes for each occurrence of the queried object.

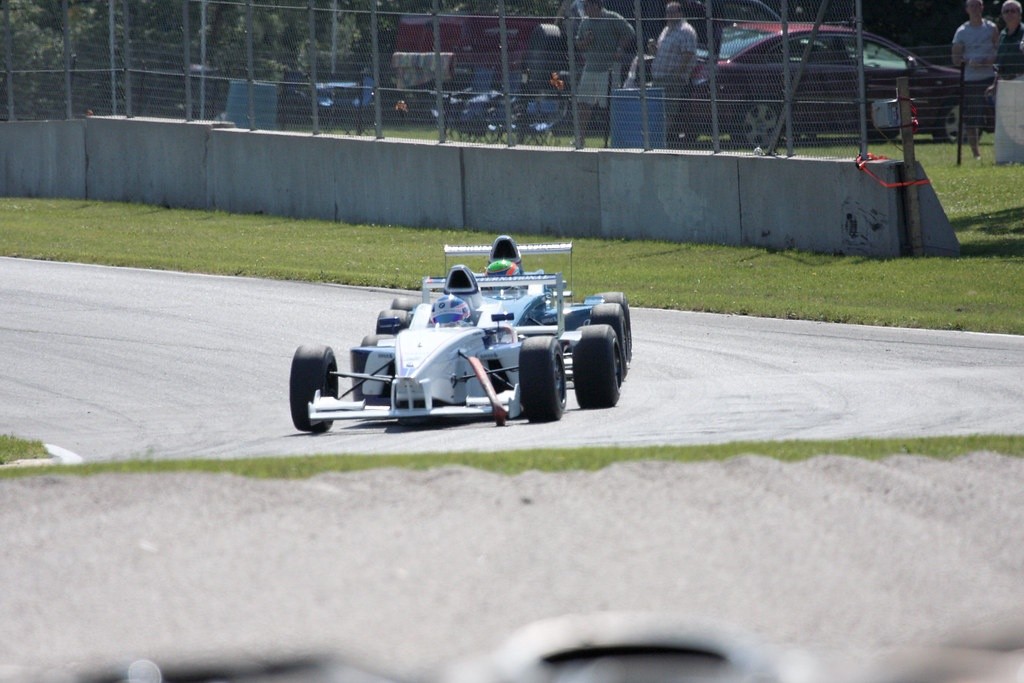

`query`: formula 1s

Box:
[289,232,633,428]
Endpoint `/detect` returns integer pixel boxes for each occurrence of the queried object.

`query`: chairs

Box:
[489,236,525,278]
[281,70,334,130]
[339,73,374,135]
[440,66,572,141]
[442,264,481,322]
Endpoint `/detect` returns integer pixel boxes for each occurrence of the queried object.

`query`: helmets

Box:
[484,260,519,290]
[431,293,471,323]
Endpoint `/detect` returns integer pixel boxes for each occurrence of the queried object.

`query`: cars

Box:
[685,20,1001,148]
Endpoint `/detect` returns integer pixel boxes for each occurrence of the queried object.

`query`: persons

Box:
[951,0,1024,161]
[553,0,698,145]
